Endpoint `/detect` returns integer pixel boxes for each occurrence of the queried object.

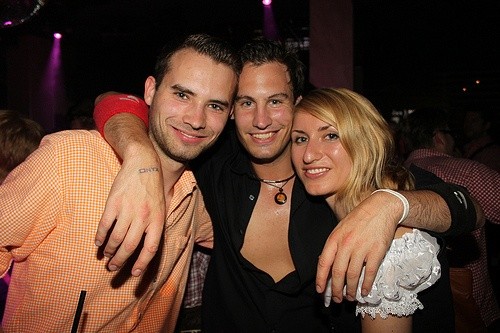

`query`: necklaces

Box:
[257,171,296,204]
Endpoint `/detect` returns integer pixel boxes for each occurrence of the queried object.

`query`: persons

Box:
[0,110,46,320]
[398,107,500,333]
[65,95,100,130]
[289,89,456,333]
[174,246,213,333]
[452,104,500,172]
[91,41,484,333]
[0,35,243,333]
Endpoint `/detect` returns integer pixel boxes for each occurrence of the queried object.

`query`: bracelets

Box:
[370,189,409,224]
